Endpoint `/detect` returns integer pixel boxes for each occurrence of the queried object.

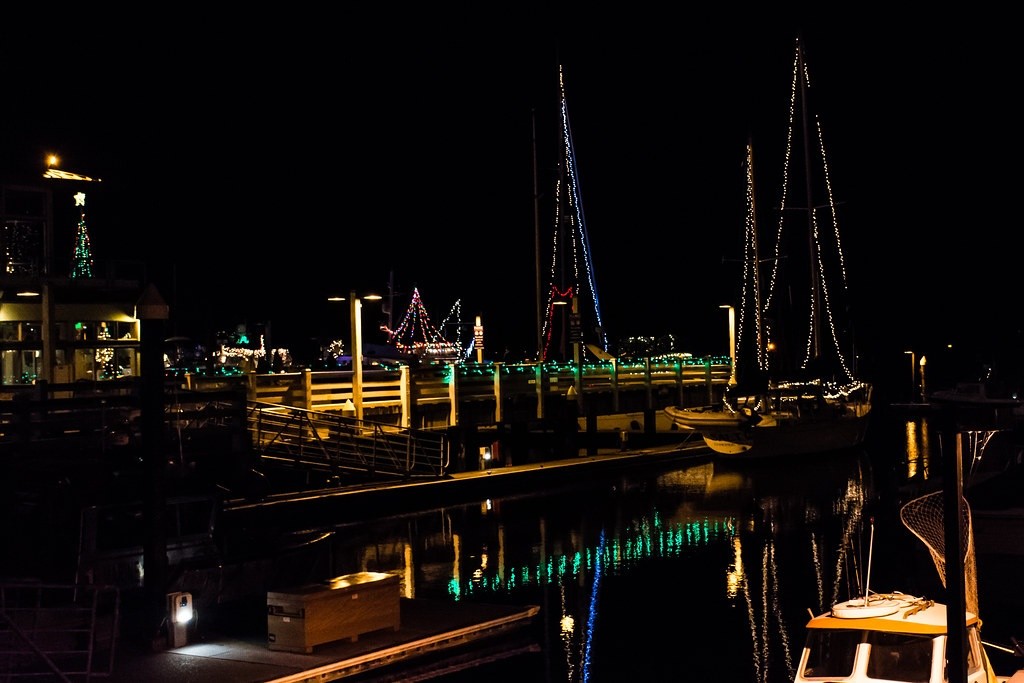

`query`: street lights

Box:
[44,154,62,417]
[719,304,737,385]
[904,349,916,387]
[328,292,383,432]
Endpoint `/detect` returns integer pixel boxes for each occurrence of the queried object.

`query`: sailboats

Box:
[663,32,884,457]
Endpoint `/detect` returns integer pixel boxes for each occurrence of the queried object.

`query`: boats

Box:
[380,288,463,370]
